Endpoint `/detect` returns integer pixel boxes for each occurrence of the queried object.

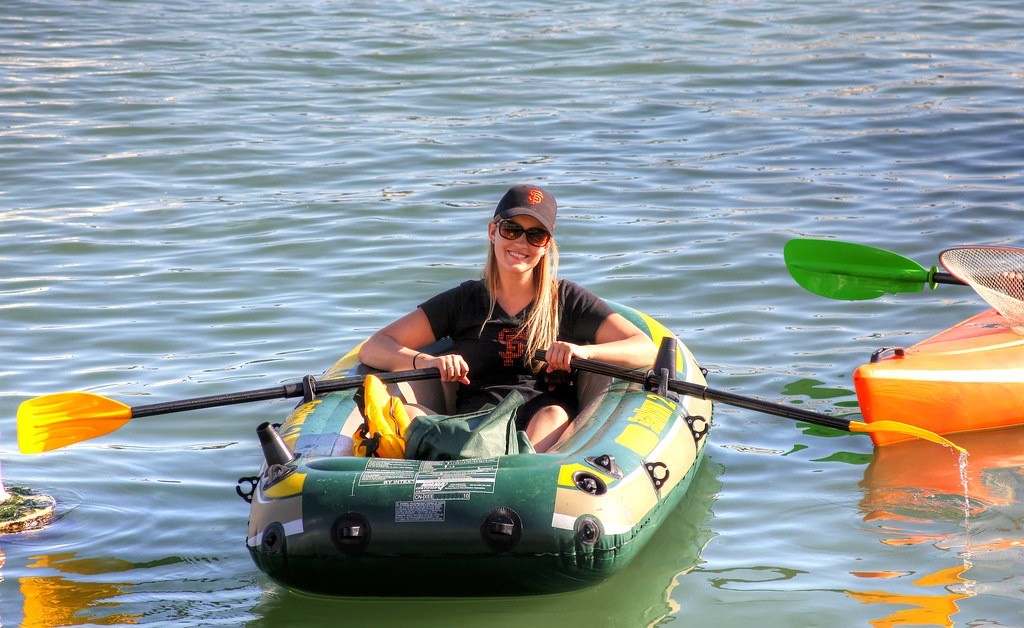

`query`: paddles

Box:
[16,365,441,458]
[782,236,1024,302]
[531,347,974,461]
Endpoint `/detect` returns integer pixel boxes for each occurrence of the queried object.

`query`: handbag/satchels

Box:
[404,386,543,461]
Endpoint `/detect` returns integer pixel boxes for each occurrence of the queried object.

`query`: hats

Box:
[494,184,557,237]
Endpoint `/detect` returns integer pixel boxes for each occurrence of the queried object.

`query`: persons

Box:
[359,184,660,453]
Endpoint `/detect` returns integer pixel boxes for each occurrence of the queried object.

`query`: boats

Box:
[233,298,714,597]
[852,308,1024,449]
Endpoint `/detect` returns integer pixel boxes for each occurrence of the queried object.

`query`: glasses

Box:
[496,220,550,247]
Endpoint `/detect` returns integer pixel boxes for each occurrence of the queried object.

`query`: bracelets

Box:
[413,353,424,369]
